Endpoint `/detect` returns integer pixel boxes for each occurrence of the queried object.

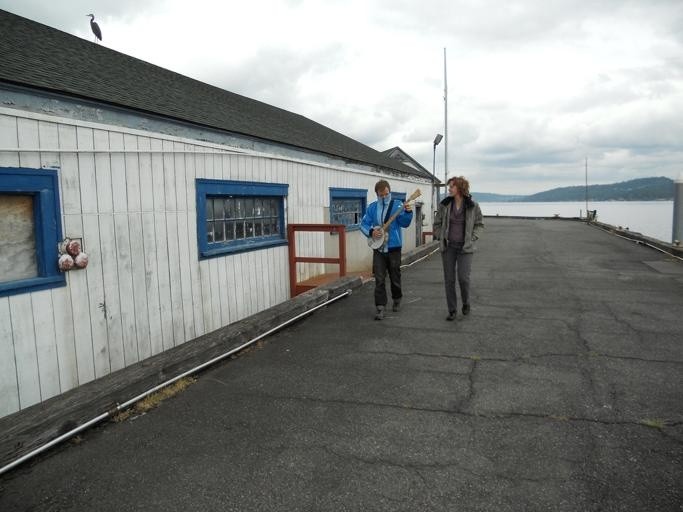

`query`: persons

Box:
[433,177,485,321]
[360,180,413,320]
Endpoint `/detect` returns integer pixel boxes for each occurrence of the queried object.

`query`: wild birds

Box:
[85,13,102,44]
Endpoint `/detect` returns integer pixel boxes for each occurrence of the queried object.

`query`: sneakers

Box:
[462,305,471,315]
[447,310,456,321]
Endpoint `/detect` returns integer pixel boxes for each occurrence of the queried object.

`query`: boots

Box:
[393,298,401,312]
[374,305,386,320]
